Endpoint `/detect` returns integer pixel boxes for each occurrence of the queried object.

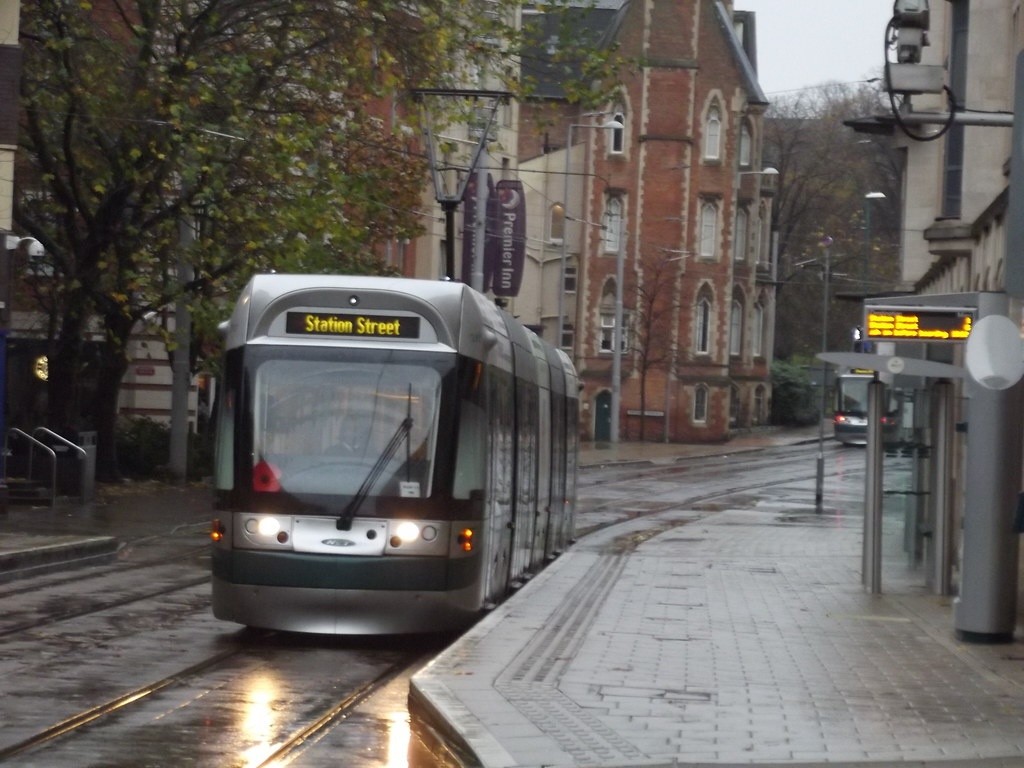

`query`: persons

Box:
[322,414,380,459]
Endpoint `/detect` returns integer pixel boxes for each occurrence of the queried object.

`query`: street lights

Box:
[407,83,509,284]
[555,121,625,351]
[724,166,779,372]
[864,190,887,298]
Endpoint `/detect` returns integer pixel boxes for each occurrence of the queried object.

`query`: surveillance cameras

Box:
[893,0,929,31]
[29,241,44,260]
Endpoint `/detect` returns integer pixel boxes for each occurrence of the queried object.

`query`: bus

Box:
[206,272,586,637]
[836,322,938,456]
